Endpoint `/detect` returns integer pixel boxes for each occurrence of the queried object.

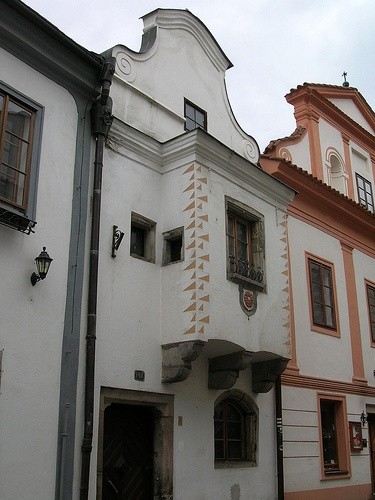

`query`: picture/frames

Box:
[349,421,363,450]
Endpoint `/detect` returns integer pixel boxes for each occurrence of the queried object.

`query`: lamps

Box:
[31,246,53,286]
